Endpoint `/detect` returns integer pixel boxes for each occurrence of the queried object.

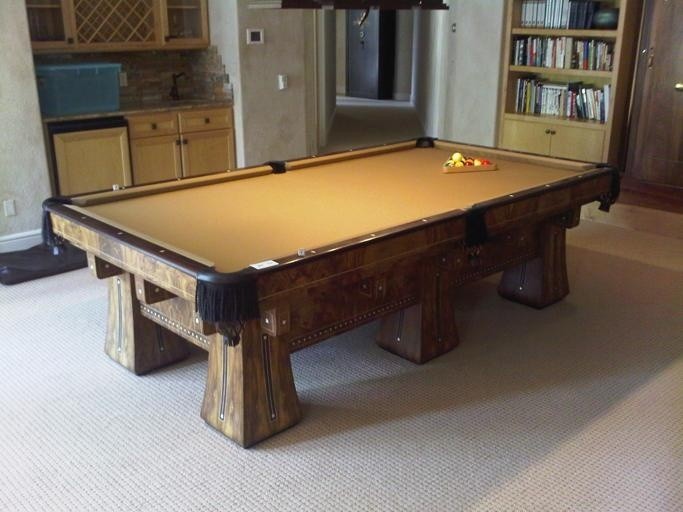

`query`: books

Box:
[33,62,122,120]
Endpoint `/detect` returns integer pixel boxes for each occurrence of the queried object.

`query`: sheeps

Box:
[172,72,185,98]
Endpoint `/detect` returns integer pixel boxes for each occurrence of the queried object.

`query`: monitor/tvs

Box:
[521,1,603,30]
[512,35,615,72]
[511,75,611,125]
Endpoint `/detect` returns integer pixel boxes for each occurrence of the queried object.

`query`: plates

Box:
[73,1,160,56]
[498,118,626,169]
[500,0,642,128]
[24,0,73,55]
[41,114,132,199]
[126,106,237,187]
[160,1,208,49]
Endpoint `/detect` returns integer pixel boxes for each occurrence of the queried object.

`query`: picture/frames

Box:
[41,135,622,449]
